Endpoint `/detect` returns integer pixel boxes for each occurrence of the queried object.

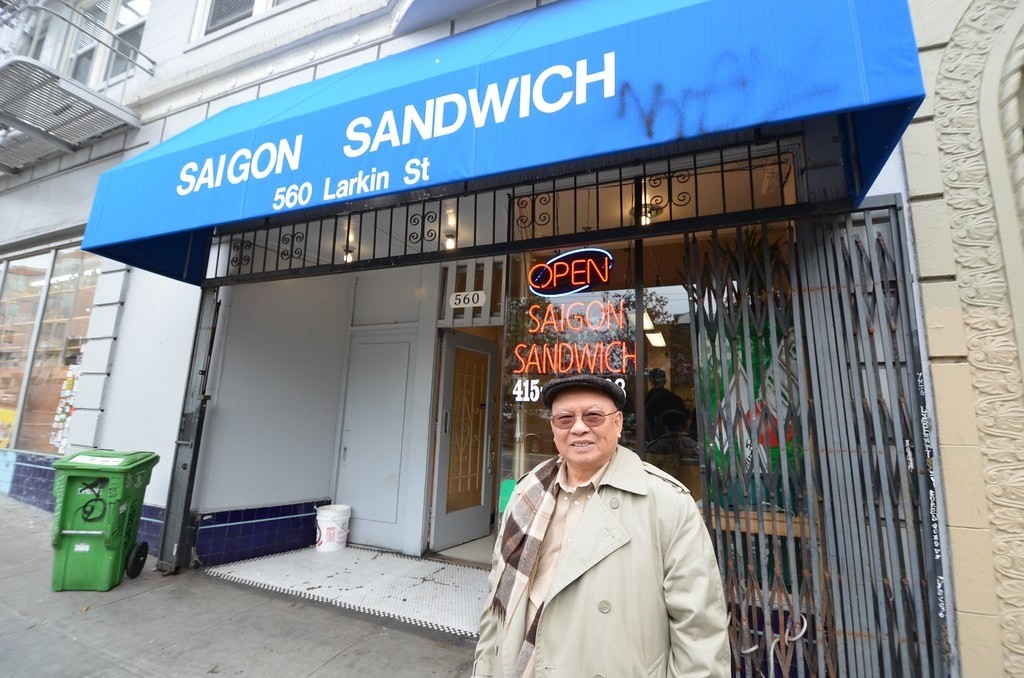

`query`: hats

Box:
[661,409,687,423]
[542,369,627,411]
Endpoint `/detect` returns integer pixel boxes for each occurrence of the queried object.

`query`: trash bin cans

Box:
[50,449,161,594]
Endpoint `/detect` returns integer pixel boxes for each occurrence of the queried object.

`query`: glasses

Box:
[547,409,618,429]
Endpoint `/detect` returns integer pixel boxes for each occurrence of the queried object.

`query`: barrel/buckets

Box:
[315,504,352,551]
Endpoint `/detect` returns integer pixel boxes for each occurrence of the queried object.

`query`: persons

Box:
[472,375,731,678]
[636,369,691,481]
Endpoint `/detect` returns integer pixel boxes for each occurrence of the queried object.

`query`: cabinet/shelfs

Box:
[697,507,839,678]
